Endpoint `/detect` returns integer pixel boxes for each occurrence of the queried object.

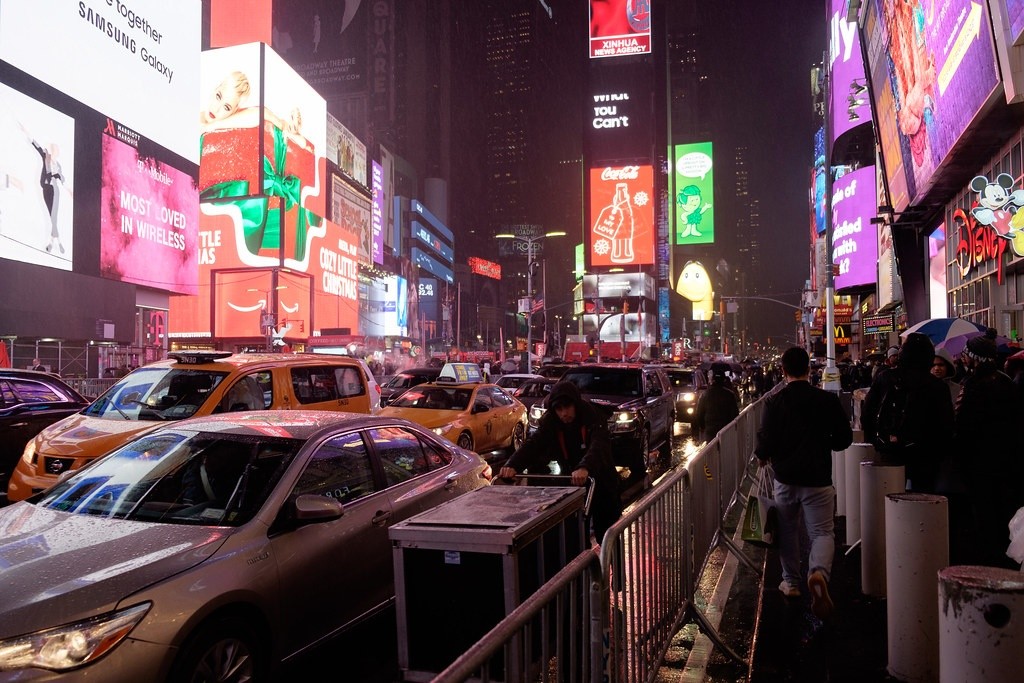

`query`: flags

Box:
[525,289,543,319]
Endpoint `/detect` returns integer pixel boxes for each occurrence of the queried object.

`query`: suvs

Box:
[526,361,677,474]
[0,368,98,510]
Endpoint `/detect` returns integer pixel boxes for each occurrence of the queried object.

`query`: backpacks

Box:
[867,368,923,463]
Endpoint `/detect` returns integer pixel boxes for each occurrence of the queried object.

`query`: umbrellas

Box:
[935,332,1010,360]
[700,360,744,372]
[899,315,989,347]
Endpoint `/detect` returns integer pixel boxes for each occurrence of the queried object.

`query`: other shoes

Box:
[611,572,626,593]
[808,573,834,619]
[778,581,801,597]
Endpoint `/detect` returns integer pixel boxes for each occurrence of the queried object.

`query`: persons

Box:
[811,354,885,399]
[32,359,46,371]
[694,358,784,404]
[264,104,306,153]
[367,361,396,376]
[752,346,854,619]
[104,364,140,378]
[336,135,354,180]
[861,328,1024,570]
[199,70,260,129]
[499,381,626,592]
[691,372,739,453]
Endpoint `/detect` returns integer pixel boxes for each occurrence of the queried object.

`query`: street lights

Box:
[495,231,565,374]
[571,268,625,363]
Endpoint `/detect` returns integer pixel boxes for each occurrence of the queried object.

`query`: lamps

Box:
[878,205,925,215]
[847,78,870,122]
[871,218,924,225]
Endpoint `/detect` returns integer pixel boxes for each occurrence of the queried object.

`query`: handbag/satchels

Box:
[741,465,777,547]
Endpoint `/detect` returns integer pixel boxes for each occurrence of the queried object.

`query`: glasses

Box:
[961,350,972,360]
[932,362,945,367]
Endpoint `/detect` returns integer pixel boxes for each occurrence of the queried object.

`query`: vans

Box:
[7,350,373,504]
[723,354,732,362]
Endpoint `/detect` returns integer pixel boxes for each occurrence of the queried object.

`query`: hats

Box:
[964,327,998,363]
[887,347,900,359]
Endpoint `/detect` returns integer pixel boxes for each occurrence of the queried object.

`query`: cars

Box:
[379,368,450,409]
[0,409,493,683]
[292,361,383,415]
[370,363,530,455]
[492,374,552,401]
[655,369,709,418]
[528,362,593,392]
